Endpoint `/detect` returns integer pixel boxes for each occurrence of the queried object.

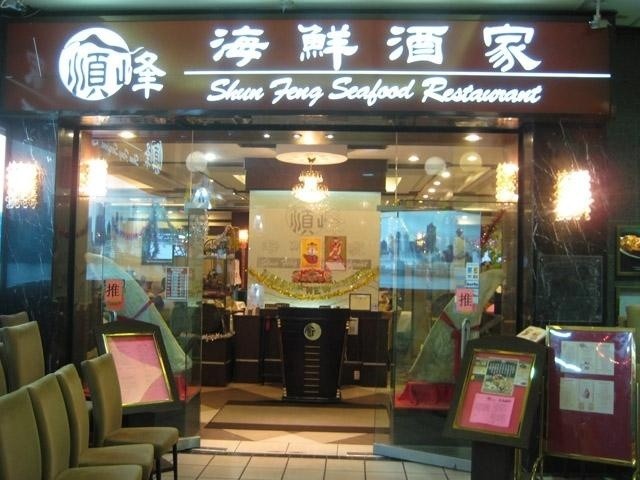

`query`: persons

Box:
[328,238,344,263]
[302,242,317,264]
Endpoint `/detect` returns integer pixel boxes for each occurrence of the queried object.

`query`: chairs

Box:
[1,312,180,480]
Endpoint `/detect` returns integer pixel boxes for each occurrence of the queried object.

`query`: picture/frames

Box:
[615,223,640,278]
[539,323,638,468]
[615,285,640,323]
[451,345,538,440]
[535,252,607,326]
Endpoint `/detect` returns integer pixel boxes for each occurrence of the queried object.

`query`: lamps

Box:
[291,170,329,204]
[3,155,46,212]
[192,188,213,206]
[494,162,518,205]
[549,168,597,224]
[84,158,108,197]
